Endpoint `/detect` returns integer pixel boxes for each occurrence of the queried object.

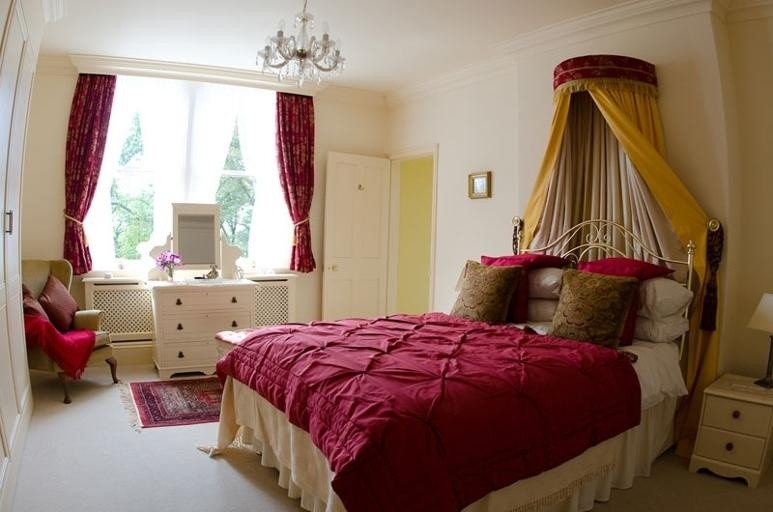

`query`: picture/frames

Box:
[465,169,492,201]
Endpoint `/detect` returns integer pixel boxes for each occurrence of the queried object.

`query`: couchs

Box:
[17,257,122,408]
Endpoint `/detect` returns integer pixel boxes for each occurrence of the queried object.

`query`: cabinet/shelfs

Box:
[146,276,260,381]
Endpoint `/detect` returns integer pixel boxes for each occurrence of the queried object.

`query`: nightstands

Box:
[686,369,772,490]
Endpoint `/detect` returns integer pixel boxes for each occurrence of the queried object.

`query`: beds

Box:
[188,212,699,511]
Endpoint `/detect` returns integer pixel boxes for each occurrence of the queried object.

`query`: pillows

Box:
[37,271,81,332]
[19,282,52,326]
[446,245,690,347]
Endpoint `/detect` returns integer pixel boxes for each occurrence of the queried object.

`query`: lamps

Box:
[249,2,350,94]
[744,289,772,390]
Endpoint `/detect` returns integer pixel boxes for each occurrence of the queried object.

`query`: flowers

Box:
[153,247,185,275]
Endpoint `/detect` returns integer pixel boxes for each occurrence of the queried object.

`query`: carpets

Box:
[116,373,228,432]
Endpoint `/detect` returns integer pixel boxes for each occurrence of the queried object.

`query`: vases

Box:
[166,269,174,282]
